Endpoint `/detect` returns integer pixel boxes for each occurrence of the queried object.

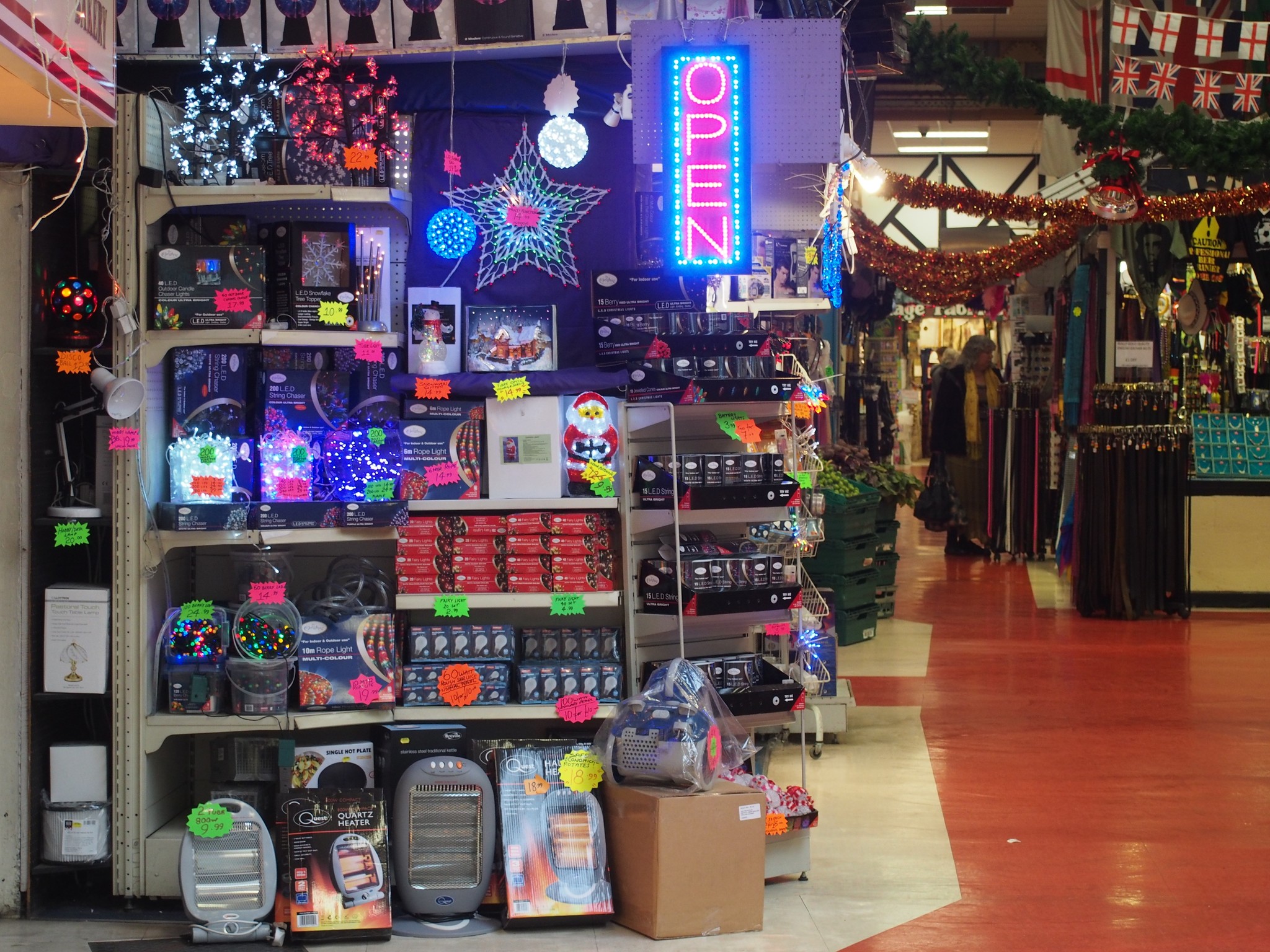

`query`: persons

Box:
[930,336,1005,554]
[809,262,824,296]
[1004,352,1012,381]
[804,314,832,445]
[773,261,796,298]
[911,346,959,409]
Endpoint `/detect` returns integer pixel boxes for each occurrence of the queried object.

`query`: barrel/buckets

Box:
[233,597,302,659]
[225,657,296,715]
[164,606,228,664]
[162,663,225,715]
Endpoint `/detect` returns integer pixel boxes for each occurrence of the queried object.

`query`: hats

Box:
[1177,279,1207,336]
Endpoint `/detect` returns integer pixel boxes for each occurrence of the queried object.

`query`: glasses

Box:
[1019,346,1052,384]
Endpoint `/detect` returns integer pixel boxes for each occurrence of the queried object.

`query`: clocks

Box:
[163,221,181,245]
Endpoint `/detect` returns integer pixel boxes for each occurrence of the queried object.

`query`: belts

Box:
[1071,383,1190,617]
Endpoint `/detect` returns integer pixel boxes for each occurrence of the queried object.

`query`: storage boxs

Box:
[45,223,902,939]
[355,227,391,331]
[779,0,914,66]
[1192,414,1270,478]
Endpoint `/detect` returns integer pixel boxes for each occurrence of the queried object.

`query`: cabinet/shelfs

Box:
[0,36,833,919]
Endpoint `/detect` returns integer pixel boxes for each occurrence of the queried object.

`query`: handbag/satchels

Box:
[913,475,947,532]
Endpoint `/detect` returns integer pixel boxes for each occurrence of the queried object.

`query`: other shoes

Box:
[945,541,958,555]
[956,539,986,556]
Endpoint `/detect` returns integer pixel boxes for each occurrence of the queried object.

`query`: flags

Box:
[1110,5,1140,45]
[1193,70,1221,110]
[1146,62,1180,101]
[1149,12,1181,53]
[1232,73,1263,113]
[1194,18,1224,57]
[1112,56,1141,95]
[1231,0,1249,11]
[1238,21,1268,61]
[1186,0,1216,8]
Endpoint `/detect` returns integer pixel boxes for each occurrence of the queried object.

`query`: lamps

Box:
[49,367,145,518]
[603,84,632,127]
[1086,177,1139,220]
[60,641,87,682]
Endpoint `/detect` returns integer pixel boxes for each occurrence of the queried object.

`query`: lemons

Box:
[812,456,860,498]
